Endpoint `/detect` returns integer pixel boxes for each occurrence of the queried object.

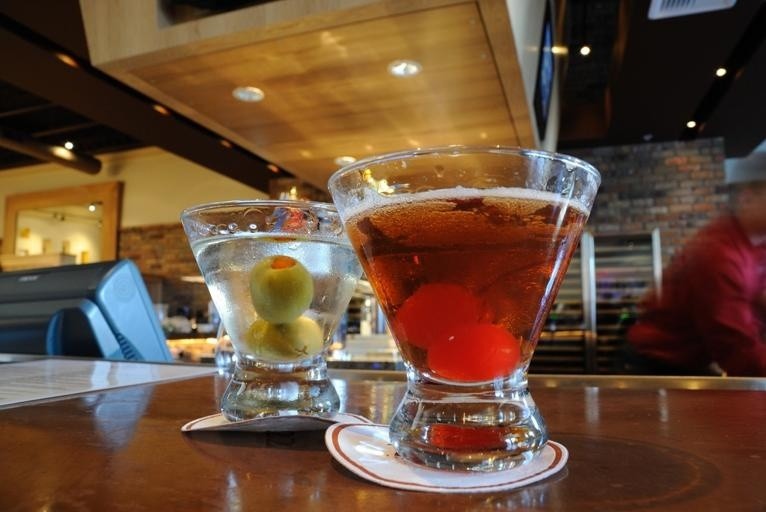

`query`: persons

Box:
[622,151,766,377]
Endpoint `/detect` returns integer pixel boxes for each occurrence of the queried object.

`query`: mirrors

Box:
[2,181,121,267]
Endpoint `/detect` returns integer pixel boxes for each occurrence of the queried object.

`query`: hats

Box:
[723,138,766,185]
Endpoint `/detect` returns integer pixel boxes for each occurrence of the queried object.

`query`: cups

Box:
[181,199,365,421]
[326,148,600,477]
